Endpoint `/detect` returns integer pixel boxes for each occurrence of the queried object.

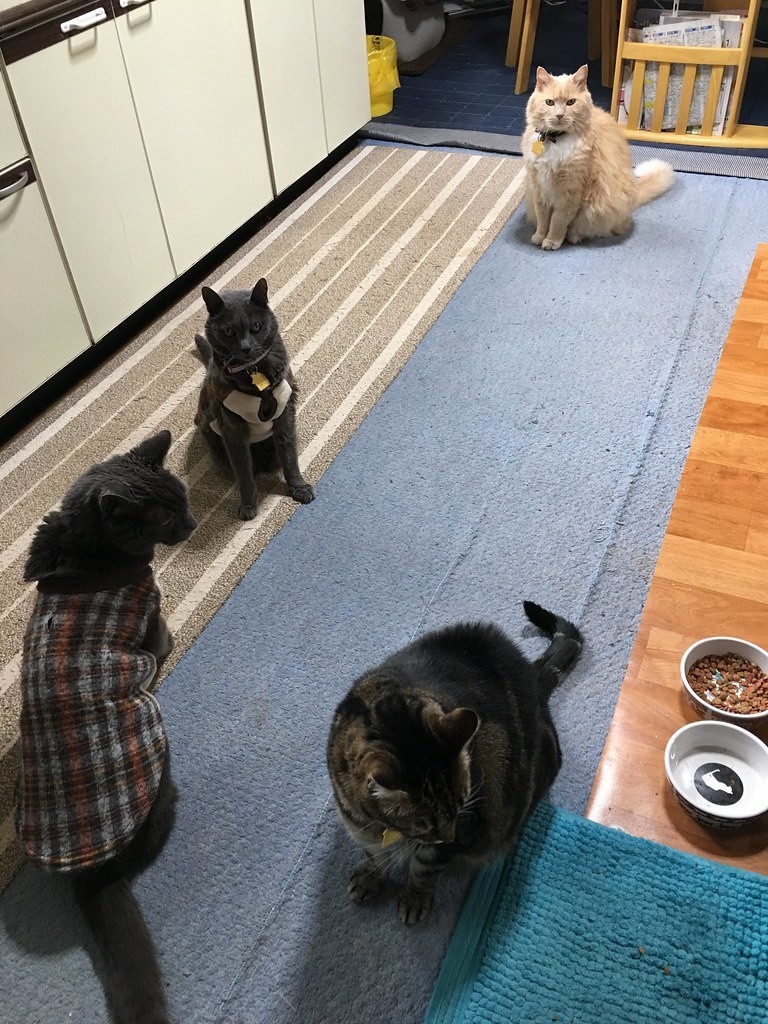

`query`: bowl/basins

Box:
[680,634,768,732]
[662,718,768,832]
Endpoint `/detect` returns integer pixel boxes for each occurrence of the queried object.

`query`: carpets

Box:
[0,163,768,1024]
[1,139,531,895]
[421,800,766,1024]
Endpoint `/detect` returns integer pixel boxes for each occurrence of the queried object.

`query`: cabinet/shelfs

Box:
[609,0,768,151]
[248,0,372,198]
[1,65,94,425]
[1,0,275,348]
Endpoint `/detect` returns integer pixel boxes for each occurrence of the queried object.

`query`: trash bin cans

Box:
[367,35,402,117]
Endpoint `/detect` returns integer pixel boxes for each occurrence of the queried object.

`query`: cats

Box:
[0,427,197,1024]
[519,63,675,251]
[193,276,317,522]
[324,600,587,926]
